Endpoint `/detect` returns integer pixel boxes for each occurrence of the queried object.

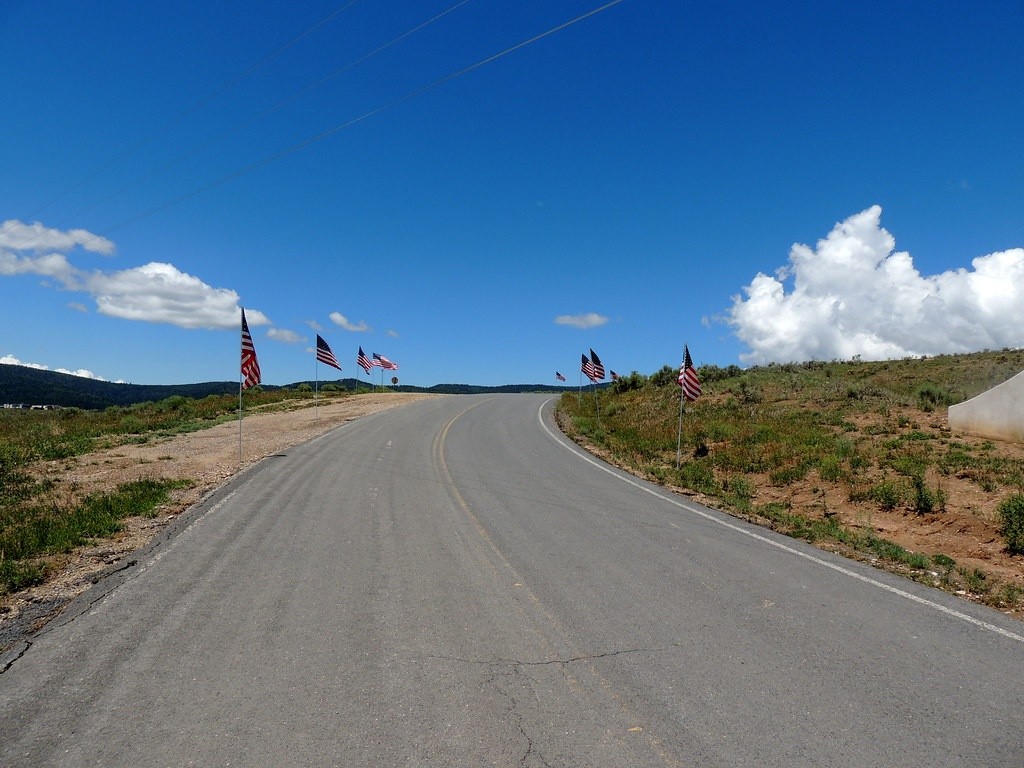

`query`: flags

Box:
[382,362,400,370]
[241,307,261,390]
[581,353,598,383]
[357,346,374,376]
[610,370,619,380]
[590,348,605,379]
[372,353,392,369]
[317,334,342,372]
[556,371,566,382]
[678,345,703,402]
